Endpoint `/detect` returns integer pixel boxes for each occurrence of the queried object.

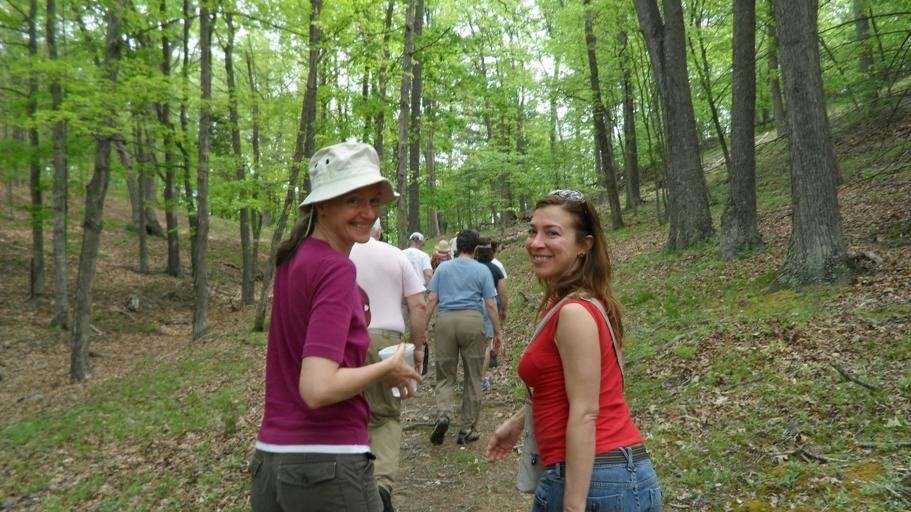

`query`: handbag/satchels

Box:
[516,399,543,493]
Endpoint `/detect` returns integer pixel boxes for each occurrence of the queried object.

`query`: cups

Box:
[377,342,419,398]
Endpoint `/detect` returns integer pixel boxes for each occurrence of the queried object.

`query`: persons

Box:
[343,215,428,510]
[426,226,510,393]
[243,140,424,512]
[409,228,506,448]
[485,188,667,510]
[398,230,434,329]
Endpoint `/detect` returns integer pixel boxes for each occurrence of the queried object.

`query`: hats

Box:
[300,142,401,212]
[433,240,452,253]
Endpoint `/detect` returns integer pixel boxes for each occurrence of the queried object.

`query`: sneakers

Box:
[429,415,449,446]
[478,375,493,392]
[378,485,396,512]
[487,348,502,369]
[458,432,480,443]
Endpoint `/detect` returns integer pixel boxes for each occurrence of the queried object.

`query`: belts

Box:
[548,445,649,468]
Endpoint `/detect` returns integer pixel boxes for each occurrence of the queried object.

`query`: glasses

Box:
[549,188,584,205]
